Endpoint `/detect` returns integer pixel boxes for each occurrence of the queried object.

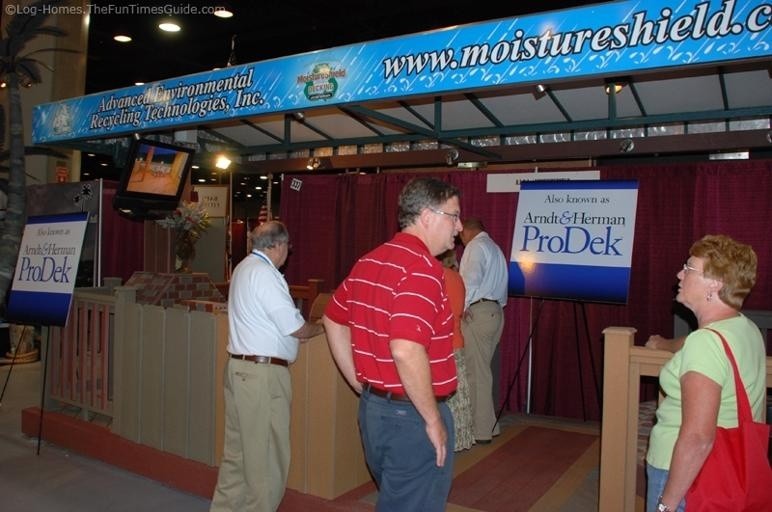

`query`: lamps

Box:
[213,143,234,174]
[443,148,460,167]
[605,81,623,97]
[617,140,634,157]
[305,153,322,172]
[533,81,547,99]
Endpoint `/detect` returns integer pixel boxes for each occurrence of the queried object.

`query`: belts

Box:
[361,385,458,402]
[470,298,498,305]
[231,355,288,367]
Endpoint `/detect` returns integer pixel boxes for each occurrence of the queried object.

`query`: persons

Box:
[209,219,326,512]
[458,217,508,443]
[643,233,765,512]
[321,175,464,512]
[438,249,478,453]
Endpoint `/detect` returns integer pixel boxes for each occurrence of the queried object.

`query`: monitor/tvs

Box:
[117,138,195,211]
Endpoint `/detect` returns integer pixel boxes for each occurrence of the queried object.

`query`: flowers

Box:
[156,196,211,257]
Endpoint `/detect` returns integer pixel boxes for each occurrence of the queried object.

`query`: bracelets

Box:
[656,495,673,512]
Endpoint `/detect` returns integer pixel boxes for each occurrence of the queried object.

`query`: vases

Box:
[172,232,197,275]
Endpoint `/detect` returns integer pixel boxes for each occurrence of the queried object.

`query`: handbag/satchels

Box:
[683,326,772,512]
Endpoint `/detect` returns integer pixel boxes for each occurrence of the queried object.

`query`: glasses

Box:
[440,211,459,224]
